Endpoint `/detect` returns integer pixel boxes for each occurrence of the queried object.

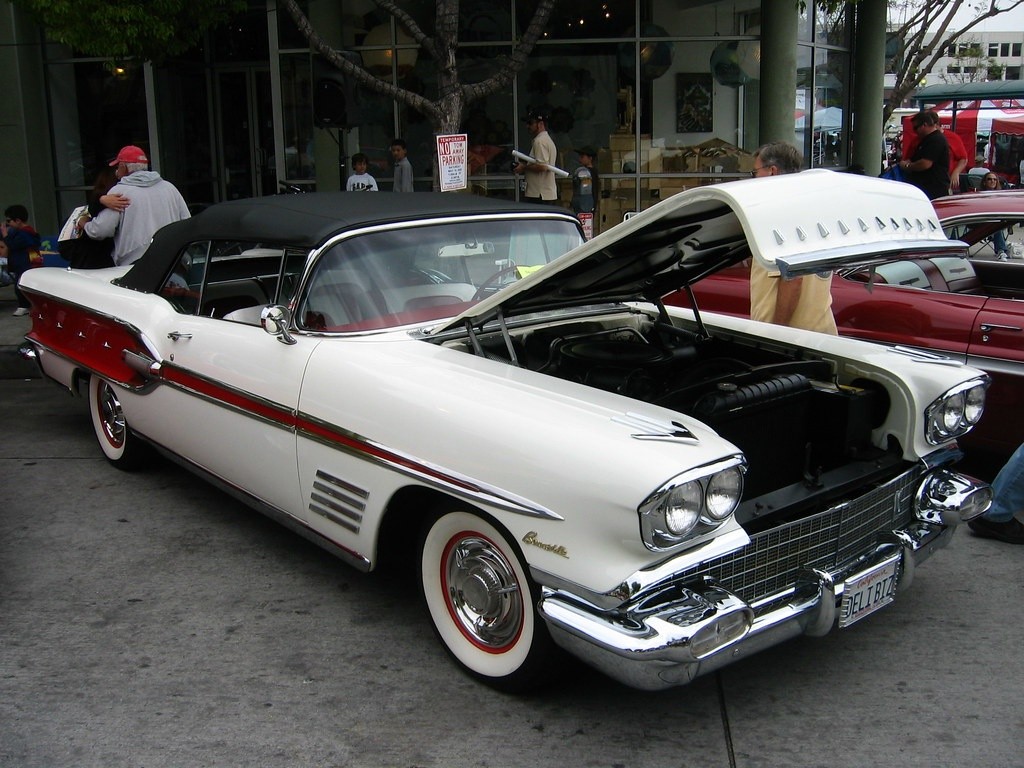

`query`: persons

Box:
[57,146,191,270]
[981,172,1008,262]
[0,204,41,316]
[900,111,968,200]
[512,111,569,204]
[983,136,1024,227]
[967,443,1024,545]
[573,146,598,219]
[751,143,838,336]
[347,153,378,191]
[392,139,413,192]
[824,135,839,166]
[969,156,990,175]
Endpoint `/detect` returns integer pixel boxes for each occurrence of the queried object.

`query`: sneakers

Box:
[13,307,30,316]
[994,253,1008,261]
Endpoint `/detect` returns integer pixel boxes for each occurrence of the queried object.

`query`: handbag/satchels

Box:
[878,162,914,181]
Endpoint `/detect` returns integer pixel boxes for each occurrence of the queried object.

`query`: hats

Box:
[520,110,549,124]
[573,145,597,160]
[109,145,148,166]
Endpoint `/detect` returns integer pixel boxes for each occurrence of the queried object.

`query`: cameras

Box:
[510,162,519,172]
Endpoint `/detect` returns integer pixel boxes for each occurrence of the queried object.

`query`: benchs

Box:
[849,257,980,294]
[173,267,369,321]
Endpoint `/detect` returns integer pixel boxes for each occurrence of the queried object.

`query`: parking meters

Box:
[573,167,593,242]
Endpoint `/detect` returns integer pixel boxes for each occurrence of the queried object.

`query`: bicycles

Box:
[217,181,309,258]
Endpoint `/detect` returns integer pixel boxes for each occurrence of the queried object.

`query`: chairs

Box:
[222,295,350,329]
[359,283,480,320]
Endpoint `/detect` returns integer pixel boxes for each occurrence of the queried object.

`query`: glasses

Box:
[6,218,13,223]
[988,178,997,182]
[913,123,924,130]
[750,164,778,178]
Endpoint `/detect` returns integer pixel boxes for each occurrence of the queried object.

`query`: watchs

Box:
[905,162,911,169]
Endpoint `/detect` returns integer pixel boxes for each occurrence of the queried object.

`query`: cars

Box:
[652,187,1024,471]
[14,165,997,697]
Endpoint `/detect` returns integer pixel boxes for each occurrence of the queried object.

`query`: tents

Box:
[795,107,854,165]
[903,79,1024,166]
